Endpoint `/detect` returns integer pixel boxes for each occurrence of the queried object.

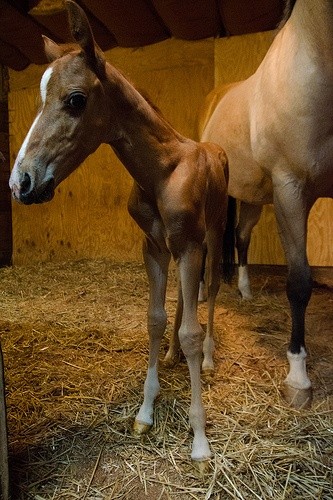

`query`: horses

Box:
[7,0,231,481]
[196,0,331,411]
